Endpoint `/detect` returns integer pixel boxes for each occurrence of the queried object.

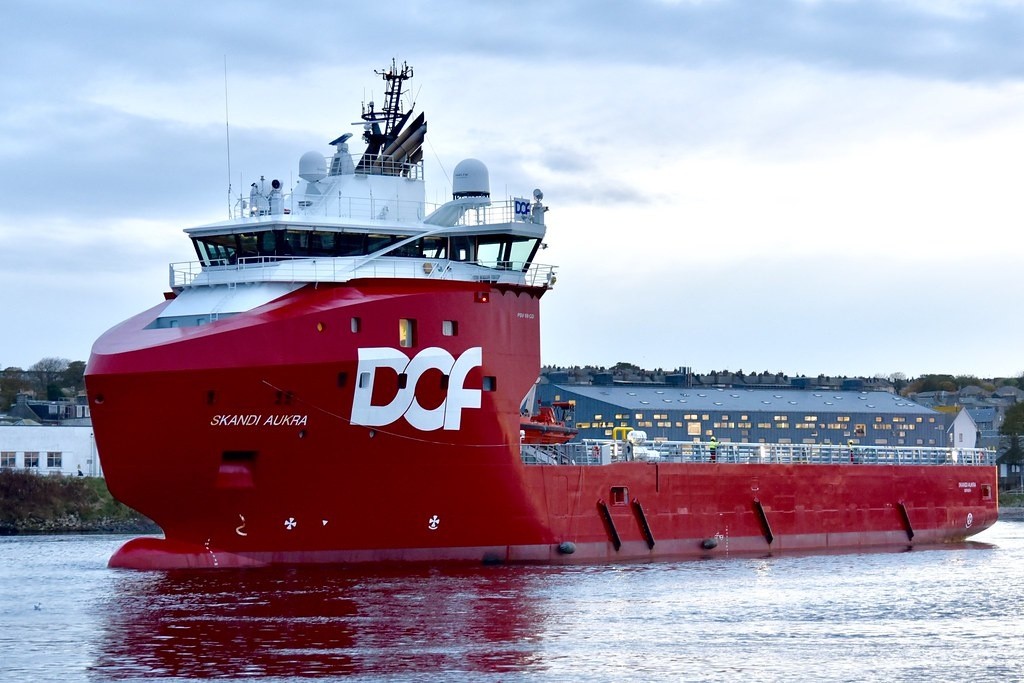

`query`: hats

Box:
[710,436,715,441]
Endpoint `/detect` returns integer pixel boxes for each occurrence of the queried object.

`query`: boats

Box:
[83,57,998,570]
[520,401,578,445]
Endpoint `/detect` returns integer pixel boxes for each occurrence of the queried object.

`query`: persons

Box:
[77,470,83,476]
[848,440,854,462]
[708,436,721,463]
